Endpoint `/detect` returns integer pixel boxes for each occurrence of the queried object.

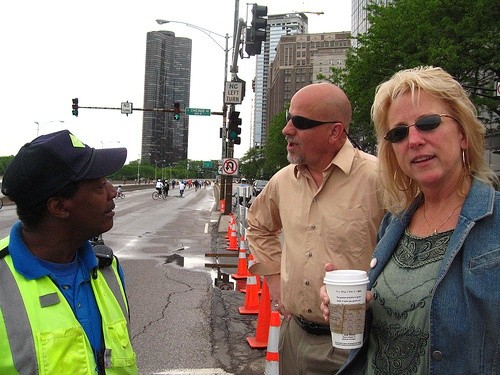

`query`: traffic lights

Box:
[244,28,263,57]
[251,2,268,41]
[72,97,79,116]
[230,111,242,144]
[173,102,180,120]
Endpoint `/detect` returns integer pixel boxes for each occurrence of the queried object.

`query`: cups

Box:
[322,269,370,349]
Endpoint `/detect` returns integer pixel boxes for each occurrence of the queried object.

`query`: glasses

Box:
[384,112,462,143]
[286,110,342,130]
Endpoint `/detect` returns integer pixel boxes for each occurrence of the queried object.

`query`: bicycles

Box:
[114,191,125,200]
[152,189,161,200]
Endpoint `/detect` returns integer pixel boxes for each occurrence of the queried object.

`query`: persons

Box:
[245,85,386,375]
[117,184,123,197]
[156,179,208,196]
[320,66,500,375]
[0,127,138,375]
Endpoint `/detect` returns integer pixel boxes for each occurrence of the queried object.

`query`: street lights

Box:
[155,159,166,179]
[138,152,152,182]
[155,0,324,201]
[33,120,65,138]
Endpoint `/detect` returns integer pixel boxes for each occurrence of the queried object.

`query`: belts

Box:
[291,315,331,337]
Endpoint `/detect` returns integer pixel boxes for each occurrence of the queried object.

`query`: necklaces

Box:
[422,202,463,235]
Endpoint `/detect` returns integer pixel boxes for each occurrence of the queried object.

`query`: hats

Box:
[1,130,127,206]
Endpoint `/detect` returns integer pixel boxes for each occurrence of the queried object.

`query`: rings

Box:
[275,304,279,307]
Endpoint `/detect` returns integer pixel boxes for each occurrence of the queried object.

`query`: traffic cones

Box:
[232,236,252,278]
[244,279,273,348]
[225,211,240,250]
[238,254,261,314]
[263,311,281,375]
